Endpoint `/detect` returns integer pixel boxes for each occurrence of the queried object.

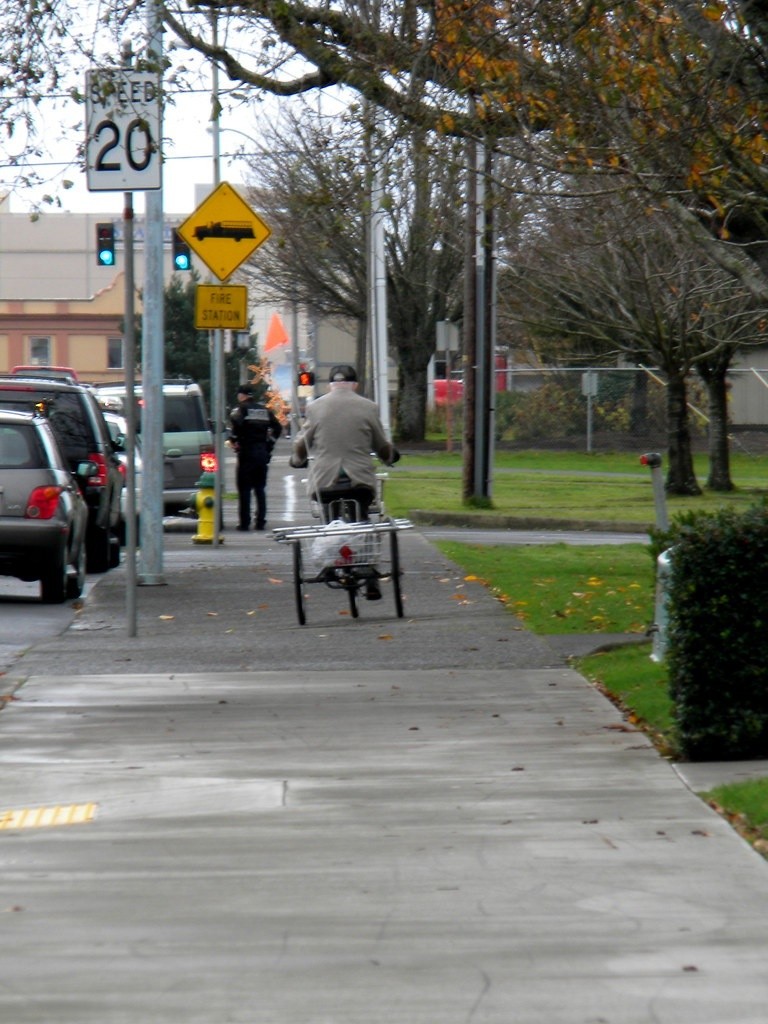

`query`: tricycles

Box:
[272,451,413,625]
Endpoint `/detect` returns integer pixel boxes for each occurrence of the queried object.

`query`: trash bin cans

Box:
[653,542,679,666]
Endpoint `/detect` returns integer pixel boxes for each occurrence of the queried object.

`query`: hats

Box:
[300,363,307,369]
[235,384,253,395]
[329,366,358,383]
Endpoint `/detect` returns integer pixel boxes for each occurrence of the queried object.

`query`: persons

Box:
[227,384,283,531]
[289,365,401,600]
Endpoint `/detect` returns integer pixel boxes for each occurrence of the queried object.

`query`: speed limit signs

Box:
[83,69,165,192]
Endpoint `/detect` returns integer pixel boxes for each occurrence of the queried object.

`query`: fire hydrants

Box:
[184,467,225,547]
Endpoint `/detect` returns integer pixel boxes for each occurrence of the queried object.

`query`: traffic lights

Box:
[172,226,190,272]
[297,371,316,386]
[97,221,113,266]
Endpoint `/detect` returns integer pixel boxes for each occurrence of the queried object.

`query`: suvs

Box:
[0,365,183,578]
[88,376,223,532]
[0,408,99,605]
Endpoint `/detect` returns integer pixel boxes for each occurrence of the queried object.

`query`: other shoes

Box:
[285,435,291,439]
[235,523,249,531]
[254,519,266,530]
[365,578,382,601]
